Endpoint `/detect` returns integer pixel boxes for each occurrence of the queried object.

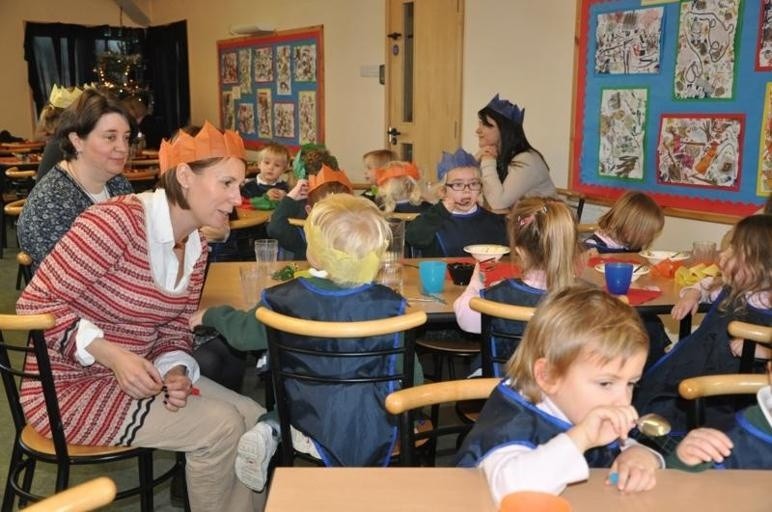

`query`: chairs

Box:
[673,372,770,429]
[694,320,770,372]
[382,378,523,467]
[4,196,34,292]
[1,310,193,510]
[253,305,438,468]
[469,294,632,379]
[1,139,590,261]
[415,329,484,384]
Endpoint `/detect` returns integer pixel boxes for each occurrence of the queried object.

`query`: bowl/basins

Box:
[637,249,690,267]
[462,244,508,262]
[595,262,650,283]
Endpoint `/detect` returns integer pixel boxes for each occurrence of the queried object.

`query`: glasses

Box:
[449,180,485,193]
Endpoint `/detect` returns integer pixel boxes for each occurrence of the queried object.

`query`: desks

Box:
[191,246,713,345]
[261,466,770,511]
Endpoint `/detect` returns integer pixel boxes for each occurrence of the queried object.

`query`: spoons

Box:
[634,411,672,439]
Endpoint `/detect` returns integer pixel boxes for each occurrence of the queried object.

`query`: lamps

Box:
[231,23,273,36]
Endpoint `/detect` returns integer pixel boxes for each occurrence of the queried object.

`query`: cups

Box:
[381,214,405,296]
[448,262,476,286]
[605,261,634,295]
[240,266,266,303]
[693,241,714,268]
[420,261,446,294]
[254,240,278,275]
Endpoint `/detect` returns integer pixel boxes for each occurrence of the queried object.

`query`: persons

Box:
[189,92,557,466]
[14,83,275,511]
[448,279,666,511]
[453,190,665,452]
[627,191,771,473]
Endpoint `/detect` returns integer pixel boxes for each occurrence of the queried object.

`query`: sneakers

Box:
[233,418,280,496]
